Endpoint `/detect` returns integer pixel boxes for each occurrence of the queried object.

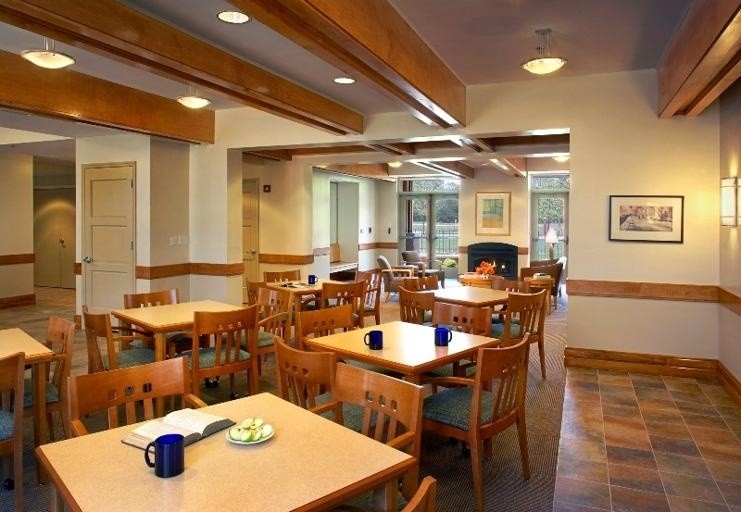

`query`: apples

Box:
[229,417,272,441]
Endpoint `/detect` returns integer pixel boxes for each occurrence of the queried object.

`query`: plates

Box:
[226,423,276,444]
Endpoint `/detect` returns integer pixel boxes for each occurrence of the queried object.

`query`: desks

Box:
[1,327,58,443]
[32,391,416,512]
[267,278,346,310]
[111,298,247,362]
[424,285,511,307]
[305,320,500,427]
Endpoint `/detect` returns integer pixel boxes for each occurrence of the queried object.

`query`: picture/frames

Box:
[474,191,511,236]
[608,194,684,244]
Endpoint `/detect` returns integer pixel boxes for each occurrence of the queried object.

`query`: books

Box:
[121,407,235,454]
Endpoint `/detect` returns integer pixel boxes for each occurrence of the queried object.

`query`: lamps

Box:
[719,176,738,227]
[545,226,561,259]
[176,84,212,109]
[521,28,569,75]
[20,37,76,69]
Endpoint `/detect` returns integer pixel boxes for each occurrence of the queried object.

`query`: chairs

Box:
[123,287,179,345]
[456,258,564,314]
[307,362,425,496]
[402,475,437,512]
[422,331,532,507]
[404,276,439,291]
[501,288,547,379]
[20,314,75,486]
[178,303,260,402]
[0,352,27,512]
[81,304,157,433]
[222,283,296,374]
[403,250,445,288]
[271,334,344,424]
[378,255,420,304]
[356,269,383,324]
[321,279,367,331]
[263,269,301,282]
[397,286,434,325]
[67,355,208,439]
[431,301,493,392]
[296,303,353,348]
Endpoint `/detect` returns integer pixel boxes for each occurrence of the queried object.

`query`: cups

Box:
[144,432,186,479]
[364,330,383,351]
[433,326,454,347]
[308,274,319,284]
